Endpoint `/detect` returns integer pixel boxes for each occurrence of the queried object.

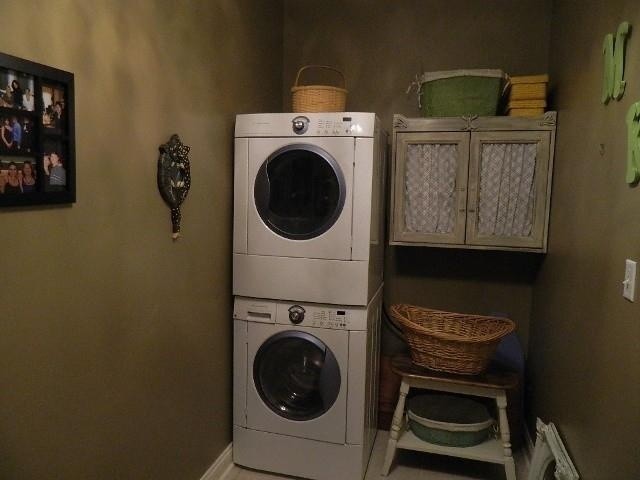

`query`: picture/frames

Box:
[0,53,78,209]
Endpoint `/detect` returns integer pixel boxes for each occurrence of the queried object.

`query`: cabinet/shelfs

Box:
[387,108,557,255]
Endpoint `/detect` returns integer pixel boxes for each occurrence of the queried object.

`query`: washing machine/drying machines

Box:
[231,112,391,306]
[231,282,386,479]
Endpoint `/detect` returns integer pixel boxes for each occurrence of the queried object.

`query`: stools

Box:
[382,352,522,480]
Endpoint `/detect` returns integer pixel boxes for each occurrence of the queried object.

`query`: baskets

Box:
[503,100,548,116]
[290,64,351,113]
[506,73,552,100]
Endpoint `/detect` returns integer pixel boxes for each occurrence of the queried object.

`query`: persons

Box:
[48,152,67,186]
[0,86,15,112]
[0,160,24,193]
[11,118,23,151]
[41,154,52,184]
[0,115,15,153]
[50,102,67,132]
[10,80,23,111]
[22,88,35,111]
[21,159,37,195]
